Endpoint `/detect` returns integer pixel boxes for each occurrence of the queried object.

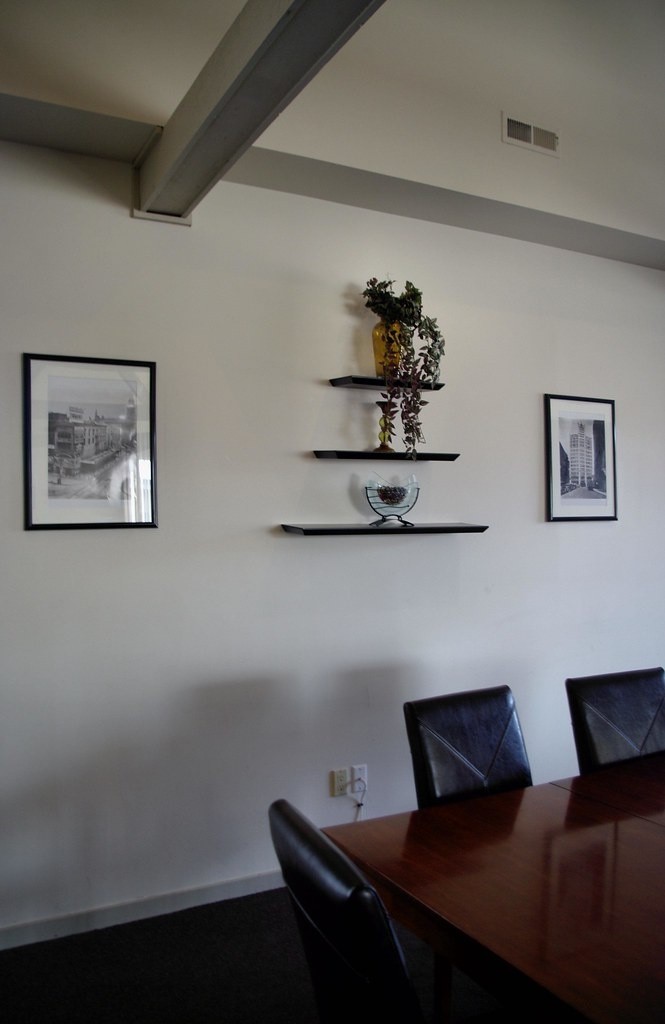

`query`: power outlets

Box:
[332,768,347,797]
[352,764,368,792]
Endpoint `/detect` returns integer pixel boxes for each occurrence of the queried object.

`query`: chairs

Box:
[404,685,533,808]
[566,667,665,774]
[268,799,426,1024]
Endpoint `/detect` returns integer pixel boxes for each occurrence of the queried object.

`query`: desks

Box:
[321,751,664,1024]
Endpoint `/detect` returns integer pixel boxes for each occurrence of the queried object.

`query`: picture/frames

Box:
[544,394,619,522]
[22,352,158,531]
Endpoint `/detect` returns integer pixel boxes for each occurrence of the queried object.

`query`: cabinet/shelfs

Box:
[282,376,490,536]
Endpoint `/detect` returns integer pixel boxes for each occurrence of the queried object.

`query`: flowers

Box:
[363,277,446,463]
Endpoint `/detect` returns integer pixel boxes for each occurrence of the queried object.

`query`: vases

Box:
[372,316,403,378]
